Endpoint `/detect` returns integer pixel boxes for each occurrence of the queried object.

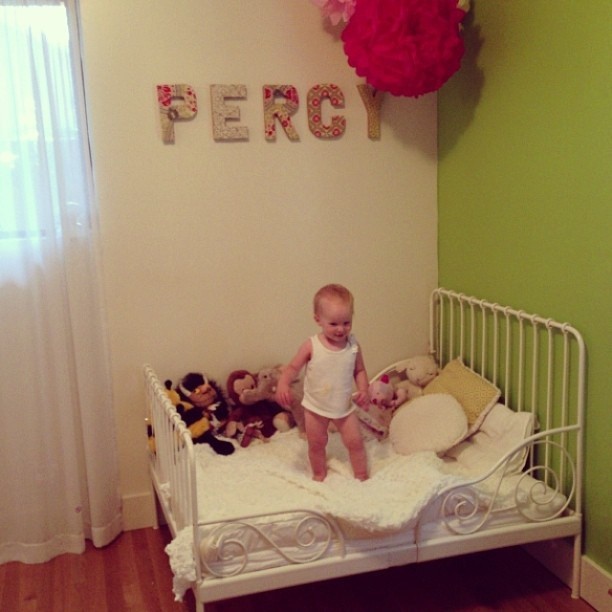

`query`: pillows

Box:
[423,356,501,440]
[387,393,467,456]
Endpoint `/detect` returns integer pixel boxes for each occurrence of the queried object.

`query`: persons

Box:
[275,284,371,482]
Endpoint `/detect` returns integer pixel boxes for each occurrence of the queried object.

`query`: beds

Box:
[143,288,587,612]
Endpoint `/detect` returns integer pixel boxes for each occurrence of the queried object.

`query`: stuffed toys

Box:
[239,365,305,430]
[395,354,438,388]
[227,370,277,438]
[175,372,228,435]
[146,380,235,455]
[369,374,411,412]
